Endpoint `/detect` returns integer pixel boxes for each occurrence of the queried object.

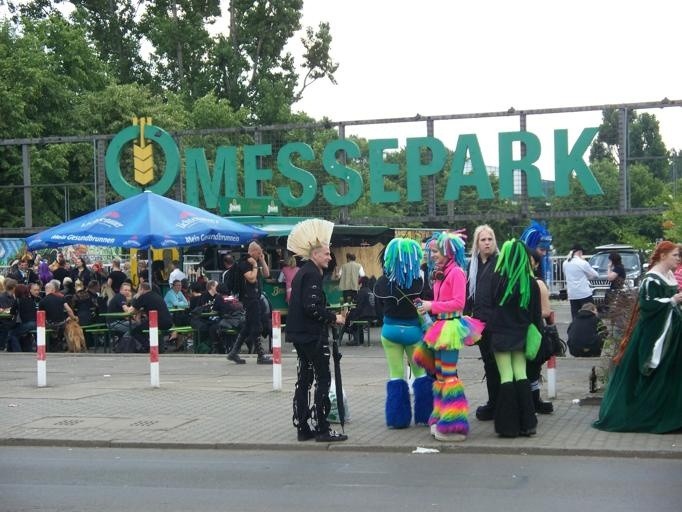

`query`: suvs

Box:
[587,249,651,316]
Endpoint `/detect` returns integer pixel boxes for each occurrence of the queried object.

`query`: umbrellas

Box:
[24,191,268,293]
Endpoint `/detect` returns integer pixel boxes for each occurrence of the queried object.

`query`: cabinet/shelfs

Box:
[179,253,204,284]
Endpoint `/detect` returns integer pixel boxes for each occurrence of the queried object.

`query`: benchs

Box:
[348,320,371,347]
[27,322,242,354]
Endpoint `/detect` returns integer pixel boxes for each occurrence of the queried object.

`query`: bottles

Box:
[589,366,598,393]
[416,302,434,329]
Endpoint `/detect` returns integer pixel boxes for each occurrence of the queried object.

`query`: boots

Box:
[254,337,273,364]
[228,333,245,364]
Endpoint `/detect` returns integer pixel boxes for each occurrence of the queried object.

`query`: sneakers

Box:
[315,431,347,441]
[476,403,495,420]
[435,433,466,440]
[431,424,437,436]
[298,430,315,440]
[535,399,553,413]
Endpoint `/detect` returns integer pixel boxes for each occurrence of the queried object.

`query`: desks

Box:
[275,308,290,317]
[1,313,15,321]
[326,303,358,345]
[99,312,136,353]
[167,307,219,322]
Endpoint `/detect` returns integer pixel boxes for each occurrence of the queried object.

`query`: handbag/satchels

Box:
[114,336,136,353]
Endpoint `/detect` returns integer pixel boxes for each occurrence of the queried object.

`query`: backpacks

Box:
[64,323,86,353]
